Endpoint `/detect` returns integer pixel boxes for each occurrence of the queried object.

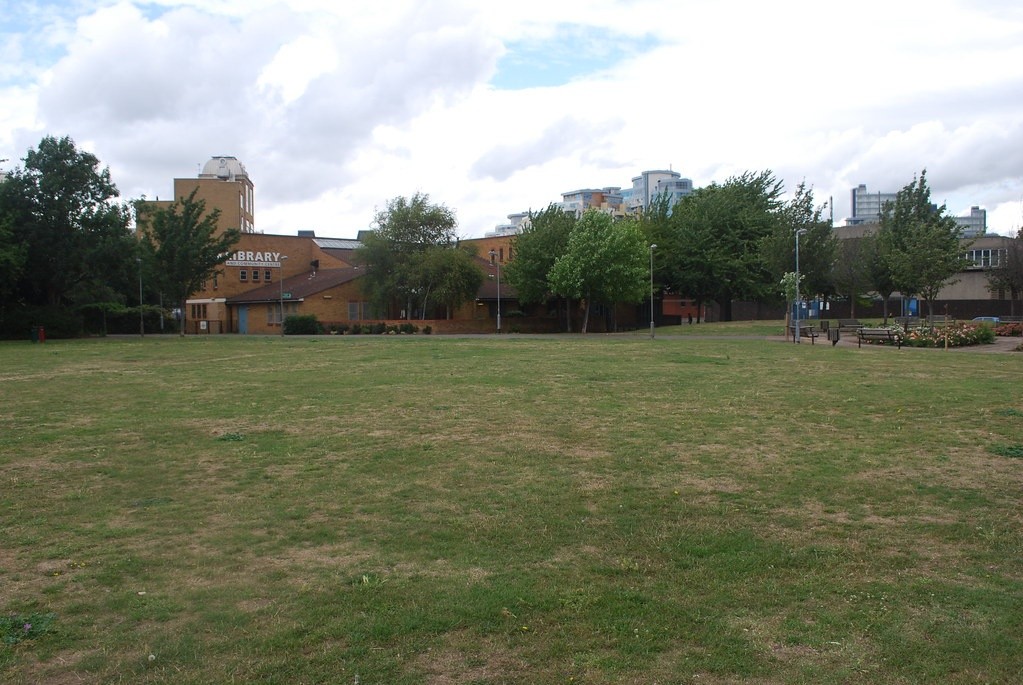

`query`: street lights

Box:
[135,258,144,338]
[795,227,808,344]
[488,251,501,334]
[279,255,288,338]
[648,243,658,340]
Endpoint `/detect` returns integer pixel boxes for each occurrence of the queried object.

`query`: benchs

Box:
[838,319,863,332]
[857,328,905,349]
[925,314,956,326]
[895,316,923,328]
[789,325,819,345]
[790,320,816,333]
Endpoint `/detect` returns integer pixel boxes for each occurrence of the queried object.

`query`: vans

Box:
[972,316,1000,322]
